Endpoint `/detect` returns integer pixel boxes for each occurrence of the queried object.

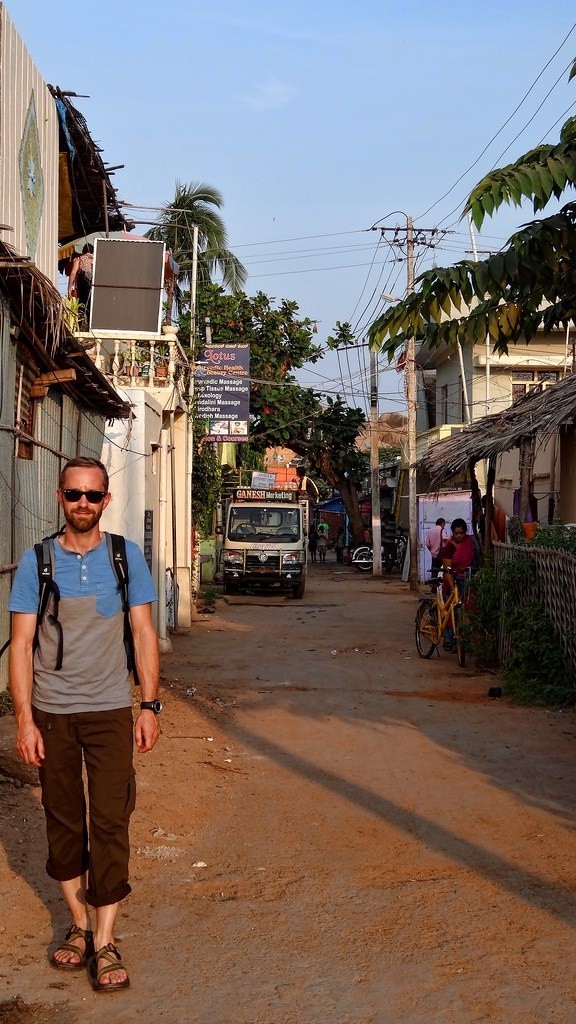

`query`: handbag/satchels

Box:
[436,547,444,562]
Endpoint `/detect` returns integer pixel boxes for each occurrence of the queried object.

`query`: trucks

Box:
[222,500,310,599]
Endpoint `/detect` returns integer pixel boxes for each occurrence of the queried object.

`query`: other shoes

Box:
[443,637,453,650]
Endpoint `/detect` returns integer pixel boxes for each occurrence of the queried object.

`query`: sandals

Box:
[53,924,93,969]
[90,944,130,991]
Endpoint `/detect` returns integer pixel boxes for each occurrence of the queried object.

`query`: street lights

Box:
[380,291,419,591]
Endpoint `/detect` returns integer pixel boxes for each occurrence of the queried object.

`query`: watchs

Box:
[140,699,163,714]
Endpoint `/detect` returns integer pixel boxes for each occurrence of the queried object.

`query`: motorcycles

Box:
[351,533,405,572]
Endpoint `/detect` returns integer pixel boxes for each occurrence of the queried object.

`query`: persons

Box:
[317,517,328,535]
[68,242,94,331]
[333,528,345,564]
[426,518,448,591]
[364,524,371,544]
[382,514,397,572]
[9,456,160,993]
[308,525,318,563]
[443,519,485,642]
[318,526,327,564]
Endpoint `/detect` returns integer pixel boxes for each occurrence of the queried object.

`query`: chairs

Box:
[277,527,294,535]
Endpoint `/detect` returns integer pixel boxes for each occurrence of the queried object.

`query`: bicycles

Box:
[414,567,473,667]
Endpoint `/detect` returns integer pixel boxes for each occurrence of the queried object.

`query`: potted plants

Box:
[463,567,498,614]
[109,345,170,378]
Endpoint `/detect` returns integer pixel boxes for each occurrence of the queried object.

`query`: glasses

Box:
[61,489,107,503]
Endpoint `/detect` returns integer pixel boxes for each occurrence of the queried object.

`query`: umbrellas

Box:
[57,232,180,281]
